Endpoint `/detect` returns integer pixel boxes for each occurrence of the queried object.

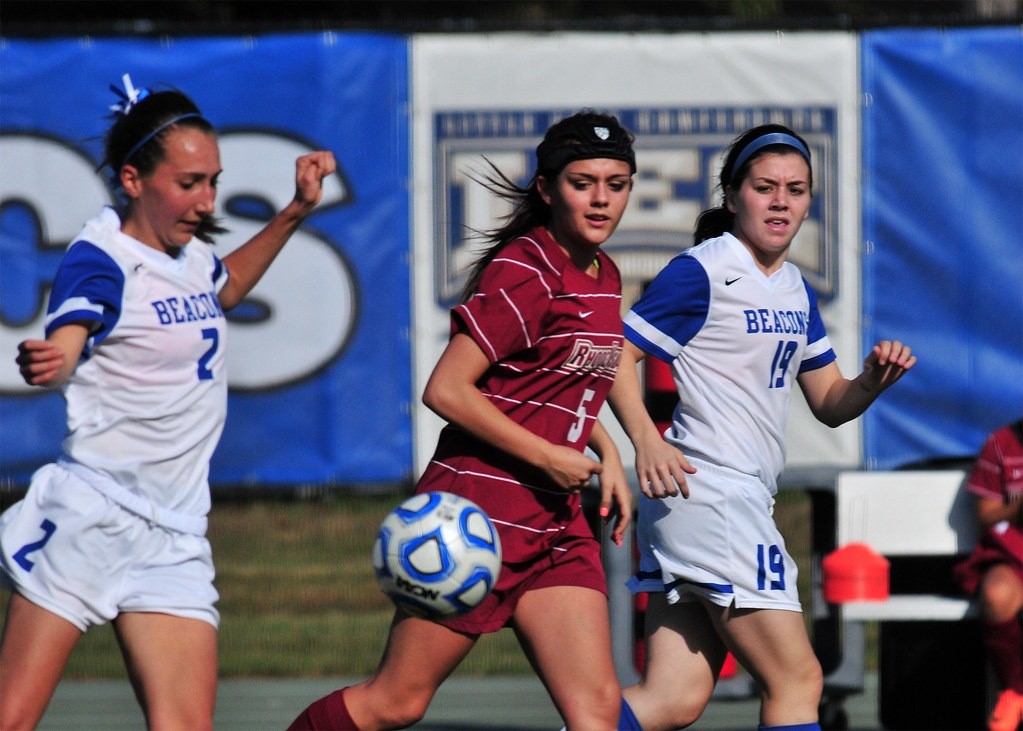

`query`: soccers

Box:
[372,491,503,620]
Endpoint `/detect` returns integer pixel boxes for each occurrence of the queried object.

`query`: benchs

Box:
[829,468,1000,731]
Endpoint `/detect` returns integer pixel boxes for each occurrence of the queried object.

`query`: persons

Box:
[952,419,1023,731]
[559,124,915,731]
[284,109,636,731]
[0,90,333,731]
